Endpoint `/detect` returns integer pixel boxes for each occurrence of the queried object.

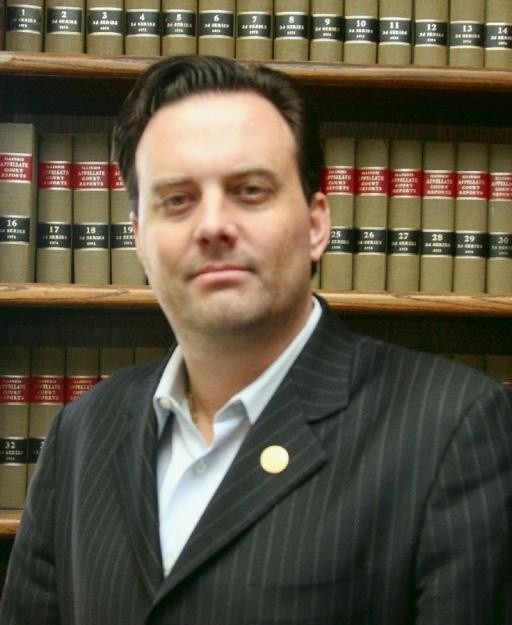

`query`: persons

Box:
[1,56,510,625]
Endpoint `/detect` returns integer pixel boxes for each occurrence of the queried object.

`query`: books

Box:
[440,351,511,386]
[1,345,165,511]
[0,108,510,297]
[1,2,511,69]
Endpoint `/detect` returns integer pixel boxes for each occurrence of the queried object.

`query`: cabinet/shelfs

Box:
[1,48,512,544]
